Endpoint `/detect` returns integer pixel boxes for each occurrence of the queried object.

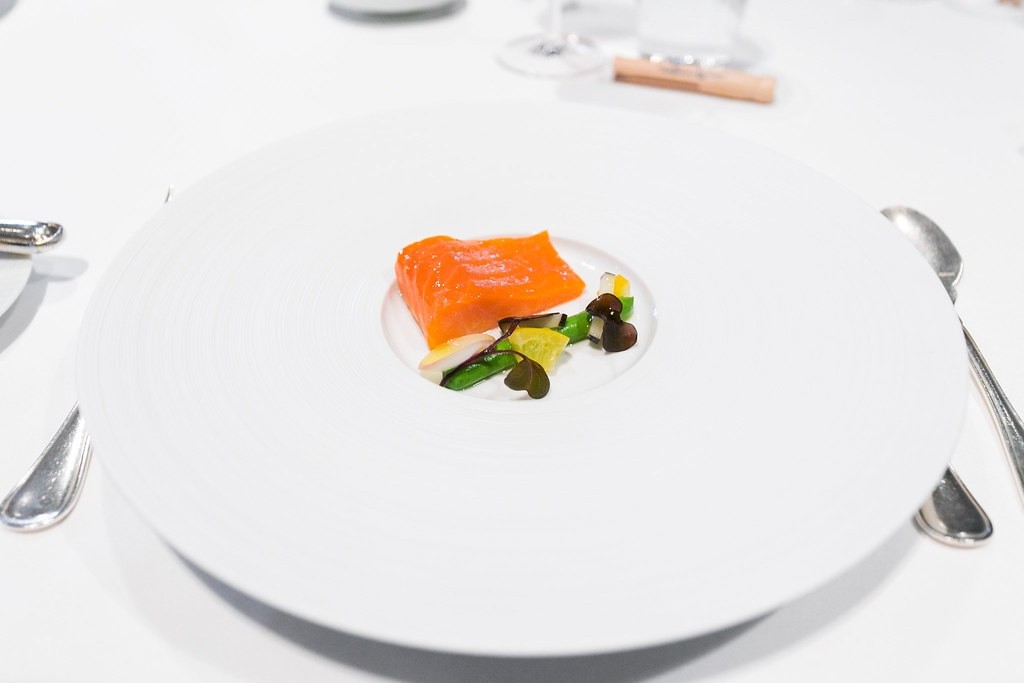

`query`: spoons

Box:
[879,205,1024,497]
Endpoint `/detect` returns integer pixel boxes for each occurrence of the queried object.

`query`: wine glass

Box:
[490,0,607,77]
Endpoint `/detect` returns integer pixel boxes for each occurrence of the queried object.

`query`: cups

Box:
[637,0,758,73]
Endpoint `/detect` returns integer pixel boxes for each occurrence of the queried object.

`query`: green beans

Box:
[442,295,634,391]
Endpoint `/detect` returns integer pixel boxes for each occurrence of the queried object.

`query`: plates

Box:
[84,95,967,658]
